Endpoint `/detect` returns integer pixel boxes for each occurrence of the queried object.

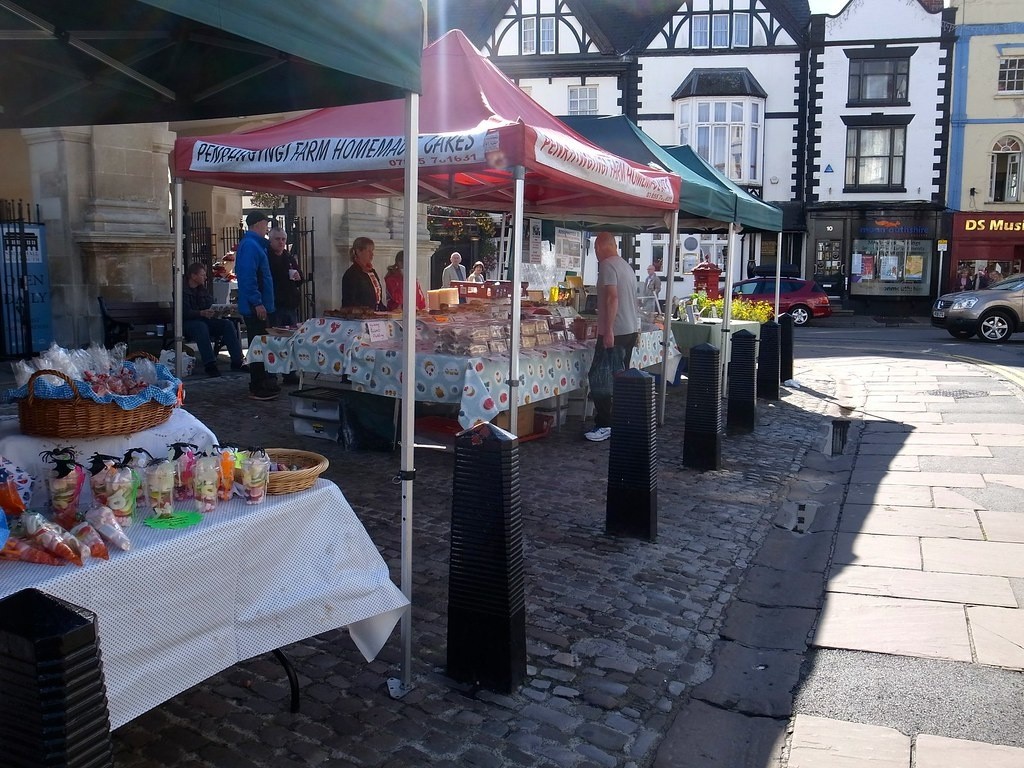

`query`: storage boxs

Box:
[493,388,597,438]
[522,217,542,264]
[289,386,353,441]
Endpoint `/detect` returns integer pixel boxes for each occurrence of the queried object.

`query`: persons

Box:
[181,262,250,377]
[342,238,388,312]
[384,251,426,310]
[467,261,484,283]
[443,252,466,288]
[955,265,1003,292]
[235,211,280,401]
[644,265,661,296]
[584,232,640,441]
[267,226,305,385]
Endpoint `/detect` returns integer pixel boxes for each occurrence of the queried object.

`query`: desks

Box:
[0,479,411,732]
[670,314,761,365]
[241,316,686,453]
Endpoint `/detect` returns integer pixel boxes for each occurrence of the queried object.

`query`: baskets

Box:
[234,448,329,496]
[18,350,179,439]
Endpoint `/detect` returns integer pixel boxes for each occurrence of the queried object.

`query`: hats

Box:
[246,211,275,226]
[473,261,484,271]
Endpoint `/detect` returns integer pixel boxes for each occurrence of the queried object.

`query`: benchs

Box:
[97,295,246,362]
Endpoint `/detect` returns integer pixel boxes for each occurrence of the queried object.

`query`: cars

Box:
[930,273,1024,343]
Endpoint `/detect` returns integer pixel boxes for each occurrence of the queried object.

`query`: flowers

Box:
[426,204,498,259]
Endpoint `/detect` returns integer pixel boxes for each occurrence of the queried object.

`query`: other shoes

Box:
[230,356,250,373]
[249,383,279,400]
[283,373,300,385]
[268,378,281,393]
[204,364,221,378]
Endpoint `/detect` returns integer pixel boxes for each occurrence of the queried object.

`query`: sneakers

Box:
[584,424,612,442]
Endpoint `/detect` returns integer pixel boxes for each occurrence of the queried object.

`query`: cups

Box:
[157,325,164,336]
[289,269,298,280]
[48,444,271,526]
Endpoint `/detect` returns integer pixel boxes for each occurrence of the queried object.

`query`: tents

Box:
[495,117,739,430]
[174,30,679,439]
[0,0,424,701]
[581,143,784,399]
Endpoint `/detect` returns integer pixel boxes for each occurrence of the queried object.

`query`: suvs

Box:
[674,265,832,328]
